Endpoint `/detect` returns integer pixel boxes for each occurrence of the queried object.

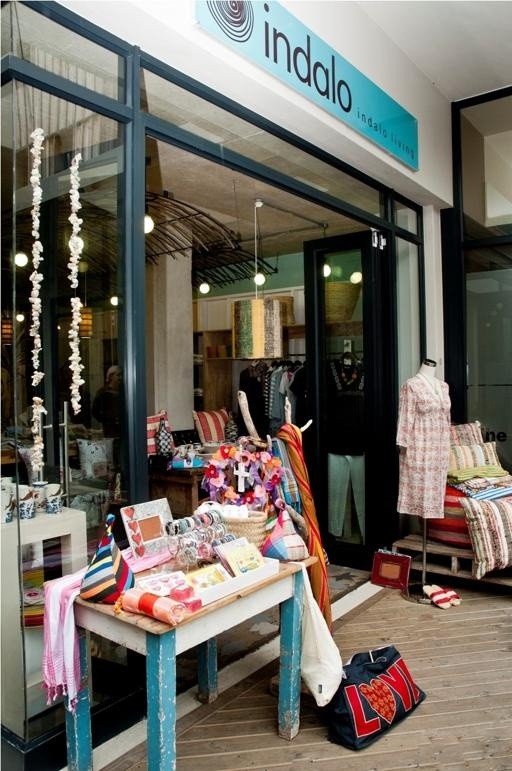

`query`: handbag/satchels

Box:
[328,645,427,751]
[288,561,347,707]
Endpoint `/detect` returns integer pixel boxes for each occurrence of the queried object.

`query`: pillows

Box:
[76,438,115,480]
[144,408,229,471]
[448,420,512,580]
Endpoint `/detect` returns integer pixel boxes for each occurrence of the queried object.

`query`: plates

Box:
[196,454,213,458]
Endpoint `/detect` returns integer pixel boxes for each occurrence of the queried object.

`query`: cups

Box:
[1,476,62,524]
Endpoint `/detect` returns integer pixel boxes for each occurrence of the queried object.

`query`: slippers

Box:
[432,584,462,606]
[423,585,451,609]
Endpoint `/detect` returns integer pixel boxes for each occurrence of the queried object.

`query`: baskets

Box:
[194,510,268,552]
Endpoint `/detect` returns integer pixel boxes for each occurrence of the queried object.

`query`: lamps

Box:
[230,198,286,362]
[0,306,12,350]
[77,254,92,340]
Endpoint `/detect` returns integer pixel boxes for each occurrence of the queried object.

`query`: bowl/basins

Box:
[203,446,218,453]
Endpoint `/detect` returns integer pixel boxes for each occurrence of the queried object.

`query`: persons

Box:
[90,365,125,469]
[394,358,452,520]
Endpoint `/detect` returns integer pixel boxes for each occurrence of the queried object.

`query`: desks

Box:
[42,556,321,770]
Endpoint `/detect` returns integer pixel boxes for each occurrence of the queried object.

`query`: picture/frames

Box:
[370,548,411,592]
[119,498,173,560]
